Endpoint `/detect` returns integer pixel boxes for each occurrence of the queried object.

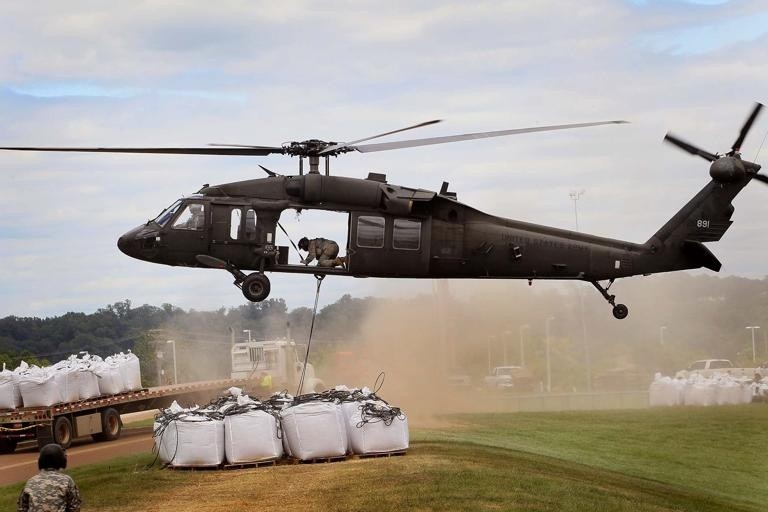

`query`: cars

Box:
[443,361,475,391]
[753,360,768,382]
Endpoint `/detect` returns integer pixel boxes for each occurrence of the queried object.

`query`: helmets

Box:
[38,444,66,469]
[298,237,309,251]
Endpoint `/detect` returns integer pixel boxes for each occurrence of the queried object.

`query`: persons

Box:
[16,443,80,511]
[173,204,203,228]
[298,236,347,269]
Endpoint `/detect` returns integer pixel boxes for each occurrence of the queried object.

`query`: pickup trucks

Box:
[676,358,757,382]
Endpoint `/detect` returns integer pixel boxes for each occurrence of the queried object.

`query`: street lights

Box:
[519,323,531,366]
[487,335,494,370]
[241,329,251,341]
[165,340,177,384]
[659,326,667,343]
[745,326,761,362]
[545,317,556,392]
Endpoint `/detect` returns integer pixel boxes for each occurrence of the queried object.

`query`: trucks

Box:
[0,339,330,451]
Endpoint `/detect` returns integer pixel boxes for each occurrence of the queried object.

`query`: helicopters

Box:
[0,103,768,318]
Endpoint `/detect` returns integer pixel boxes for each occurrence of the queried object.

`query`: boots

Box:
[333,256,346,268]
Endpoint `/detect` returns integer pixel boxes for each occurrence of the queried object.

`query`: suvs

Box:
[482,366,525,391]
